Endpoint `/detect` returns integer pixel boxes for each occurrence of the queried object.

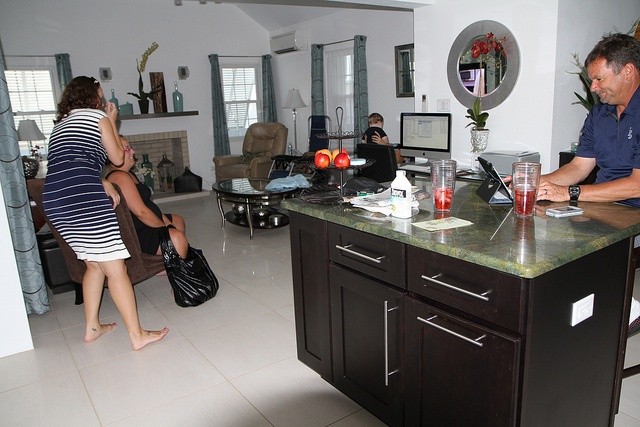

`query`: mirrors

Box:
[447,19,520,111]
[393,42,414,97]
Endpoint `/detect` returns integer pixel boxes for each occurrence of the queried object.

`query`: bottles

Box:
[391,169,412,220]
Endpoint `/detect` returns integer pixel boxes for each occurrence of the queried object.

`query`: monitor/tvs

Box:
[400,112,452,173]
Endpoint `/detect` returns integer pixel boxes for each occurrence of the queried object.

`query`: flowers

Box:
[484,31,512,83]
[465,38,491,129]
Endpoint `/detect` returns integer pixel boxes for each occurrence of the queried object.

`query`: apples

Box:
[314,148,350,170]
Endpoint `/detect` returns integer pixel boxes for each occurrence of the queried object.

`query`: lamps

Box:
[280,89,308,150]
[18,120,46,157]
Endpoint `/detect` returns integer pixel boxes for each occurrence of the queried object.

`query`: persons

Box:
[103,134,189,276]
[363,131,411,188]
[361,113,405,164]
[501,32,640,249]
[42,75,168,351]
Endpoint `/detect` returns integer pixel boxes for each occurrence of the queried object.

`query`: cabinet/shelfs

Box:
[288,210,332,382]
[329,223,530,426]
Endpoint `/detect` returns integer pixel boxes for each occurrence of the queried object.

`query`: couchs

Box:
[213,122,288,178]
[25,178,164,305]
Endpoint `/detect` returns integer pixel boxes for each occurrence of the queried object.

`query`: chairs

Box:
[268,115,331,179]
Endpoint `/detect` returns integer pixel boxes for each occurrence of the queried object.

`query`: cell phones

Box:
[545,205,584,219]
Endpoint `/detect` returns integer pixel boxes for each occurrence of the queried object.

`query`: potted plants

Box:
[126,42,163,114]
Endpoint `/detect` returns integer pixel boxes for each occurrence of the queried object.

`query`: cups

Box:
[512,162,541,219]
[432,159,456,213]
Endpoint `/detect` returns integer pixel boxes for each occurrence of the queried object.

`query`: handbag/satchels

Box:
[159,226,219,307]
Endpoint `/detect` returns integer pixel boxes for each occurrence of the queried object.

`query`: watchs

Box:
[568,185,581,205]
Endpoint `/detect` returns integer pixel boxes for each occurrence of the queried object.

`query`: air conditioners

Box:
[269,29,304,54]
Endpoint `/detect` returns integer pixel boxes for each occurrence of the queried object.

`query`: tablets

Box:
[477,156,514,201]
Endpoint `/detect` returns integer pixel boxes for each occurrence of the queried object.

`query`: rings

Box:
[544,189,548,197]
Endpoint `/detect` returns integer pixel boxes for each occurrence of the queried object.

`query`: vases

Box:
[142,154,154,191]
[470,129,489,153]
